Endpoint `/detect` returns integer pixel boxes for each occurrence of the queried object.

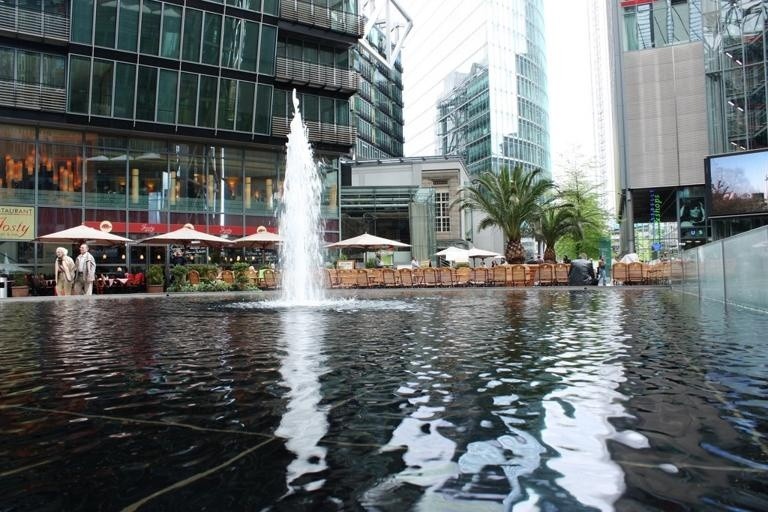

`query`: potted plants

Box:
[11,274,30,296]
[145,264,164,292]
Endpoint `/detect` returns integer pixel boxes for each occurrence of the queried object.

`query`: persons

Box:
[74,243,97,295]
[409,251,670,272]
[567,254,598,286]
[54,247,76,296]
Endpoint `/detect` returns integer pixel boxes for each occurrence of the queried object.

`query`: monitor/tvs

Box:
[703,147,767,219]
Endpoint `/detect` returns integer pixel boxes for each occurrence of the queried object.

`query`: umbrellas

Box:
[229,231,285,261]
[28,224,134,245]
[432,244,505,268]
[322,232,411,262]
[136,227,234,259]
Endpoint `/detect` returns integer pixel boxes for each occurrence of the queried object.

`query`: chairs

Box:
[187,262,571,288]
[613,263,628,287]
[627,262,648,285]
